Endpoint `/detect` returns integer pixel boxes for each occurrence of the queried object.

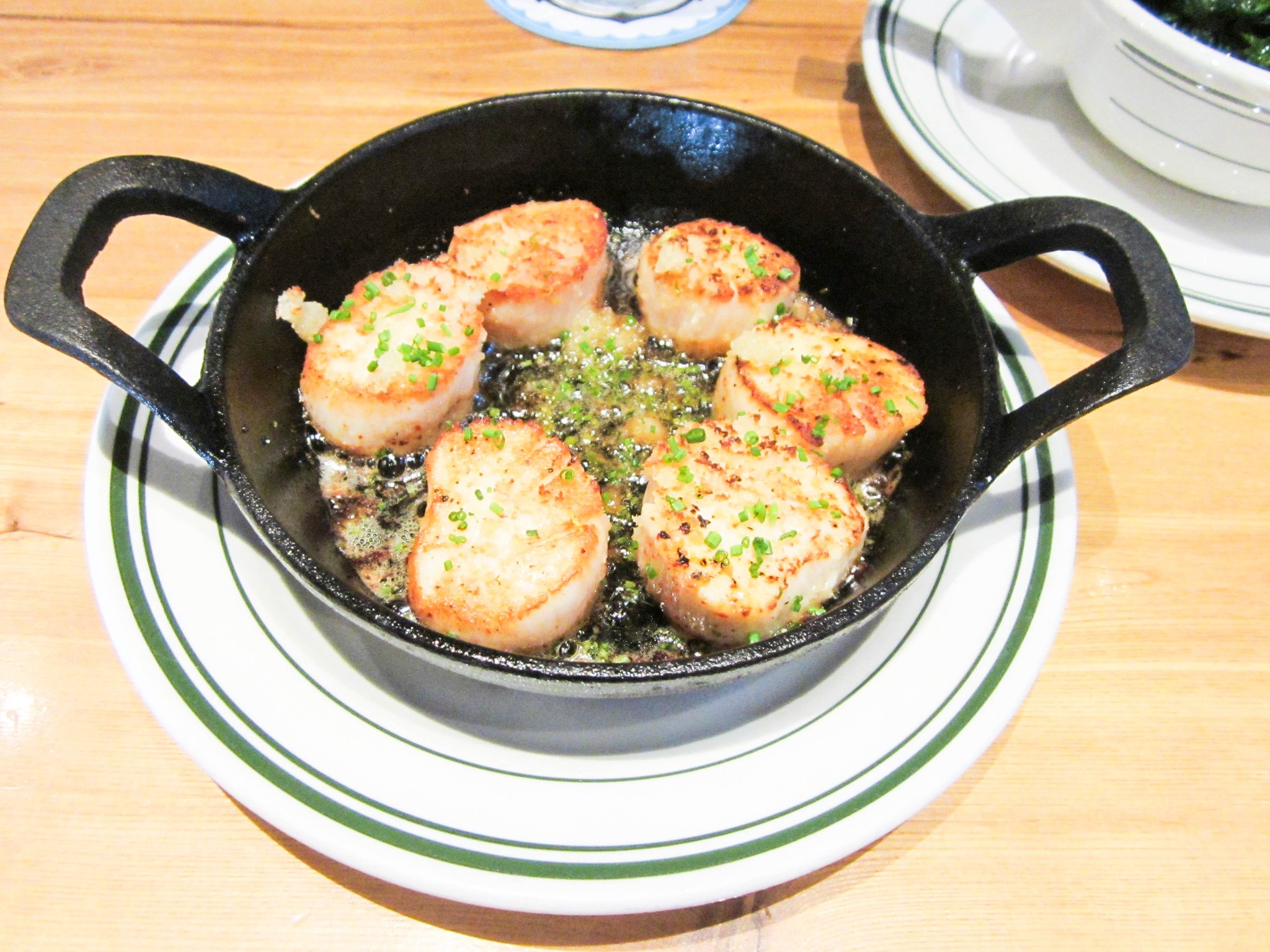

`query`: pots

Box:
[2,89,1195,698]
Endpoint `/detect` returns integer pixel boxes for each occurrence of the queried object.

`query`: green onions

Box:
[311,241,917,649]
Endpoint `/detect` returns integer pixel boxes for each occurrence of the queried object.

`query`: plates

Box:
[487,0,754,50]
[860,0,1270,342]
[83,174,1077,915]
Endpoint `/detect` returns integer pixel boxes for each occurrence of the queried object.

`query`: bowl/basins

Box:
[1063,0,1270,209]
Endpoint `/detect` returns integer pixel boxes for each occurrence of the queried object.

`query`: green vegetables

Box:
[1145,0,1270,70]
